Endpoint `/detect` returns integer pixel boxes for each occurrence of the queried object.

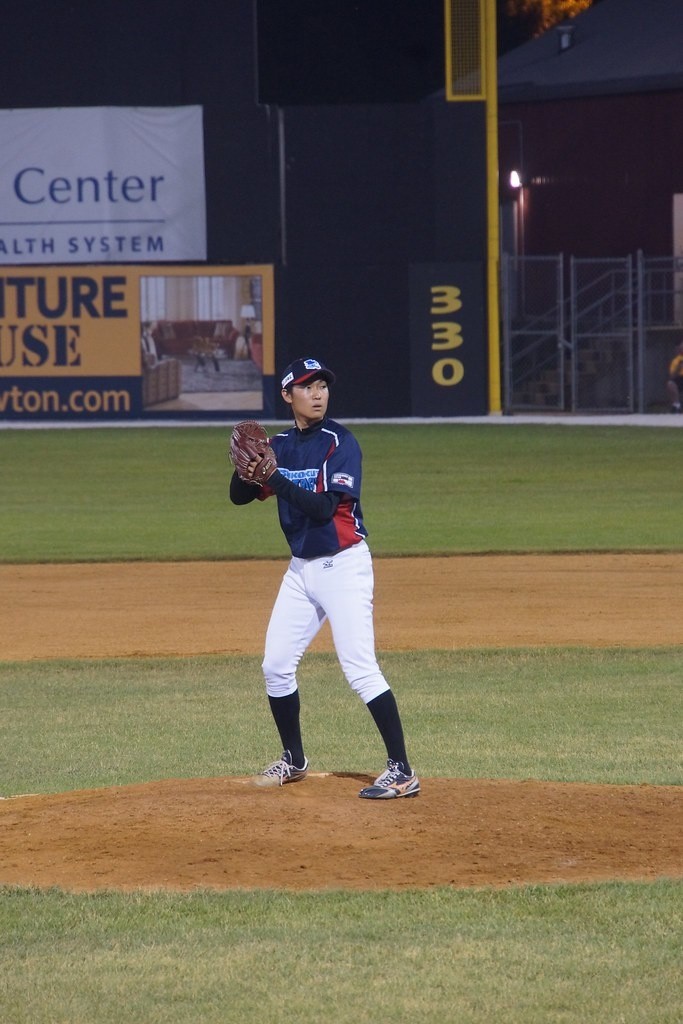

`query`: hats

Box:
[281,358,336,389]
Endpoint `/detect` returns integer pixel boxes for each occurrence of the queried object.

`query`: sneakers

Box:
[358,758,420,798]
[253,749,308,787]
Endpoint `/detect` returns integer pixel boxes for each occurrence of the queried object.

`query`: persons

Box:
[229,357,421,800]
[141,315,169,362]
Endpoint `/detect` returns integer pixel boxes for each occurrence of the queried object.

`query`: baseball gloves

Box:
[227,418,279,491]
